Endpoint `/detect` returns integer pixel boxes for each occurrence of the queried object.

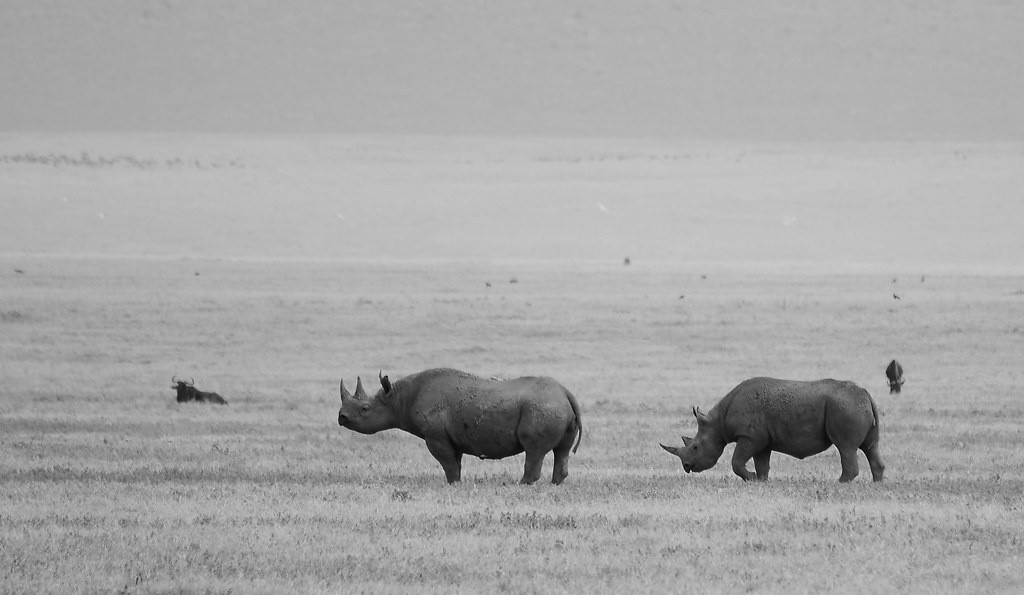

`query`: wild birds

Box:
[484,254,926,302]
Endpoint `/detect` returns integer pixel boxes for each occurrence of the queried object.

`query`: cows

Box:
[171,376,227,402]
[885,360,906,394]
